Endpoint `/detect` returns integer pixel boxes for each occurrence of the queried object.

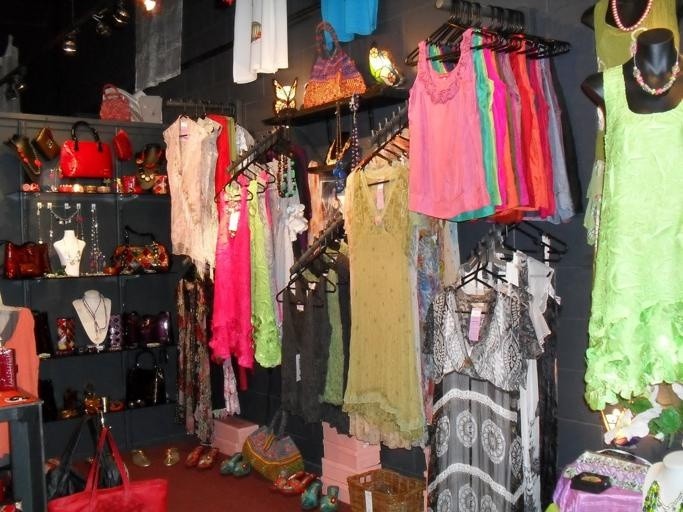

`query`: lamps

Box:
[0,62,31,105]
[62,0,158,53]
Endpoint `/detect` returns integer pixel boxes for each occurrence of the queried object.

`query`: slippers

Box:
[319,485,339,512]
[270,471,305,492]
[281,473,316,495]
[300,479,322,509]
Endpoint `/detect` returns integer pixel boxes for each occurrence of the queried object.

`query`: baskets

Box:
[346,468,426,512]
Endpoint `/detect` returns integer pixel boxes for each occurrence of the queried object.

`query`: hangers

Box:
[213,127,295,203]
[176,96,237,139]
[275,114,410,308]
[403,1,572,68]
[443,218,568,315]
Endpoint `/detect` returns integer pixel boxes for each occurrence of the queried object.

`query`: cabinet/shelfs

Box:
[0,190,193,465]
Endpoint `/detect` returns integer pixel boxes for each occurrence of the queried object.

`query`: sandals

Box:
[198,448,218,471]
[186,445,205,467]
[234,456,251,477]
[220,452,242,475]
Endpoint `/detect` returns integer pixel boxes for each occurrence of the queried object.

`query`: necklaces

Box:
[81,294,108,343]
[10,138,42,176]
[135,145,164,182]
[631,50,680,96]
[36,199,102,267]
[333,101,347,194]
[349,93,360,172]
[612,0,652,31]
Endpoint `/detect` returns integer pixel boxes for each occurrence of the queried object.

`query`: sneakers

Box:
[165,447,180,466]
[132,448,150,468]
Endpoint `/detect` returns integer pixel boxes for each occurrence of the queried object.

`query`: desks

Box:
[0,384,49,512]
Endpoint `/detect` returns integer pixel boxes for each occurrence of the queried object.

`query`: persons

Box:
[580,0,683,250]
[580,26,683,414]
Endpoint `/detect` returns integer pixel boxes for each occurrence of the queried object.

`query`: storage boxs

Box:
[205,414,384,508]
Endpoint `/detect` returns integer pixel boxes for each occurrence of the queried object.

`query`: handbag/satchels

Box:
[111,129,134,162]
[31,126,60,161]
[107,224,169,275]
[0,239,53,280]
[0,337,18,391]
[46,415,127,498]
[99,84,132,121]
[123,310,175,345]
[126,351,168,406]
[303,22,367,109]
[58,120,114,179]
[48,428,170,512]
[242,410,304,480]
